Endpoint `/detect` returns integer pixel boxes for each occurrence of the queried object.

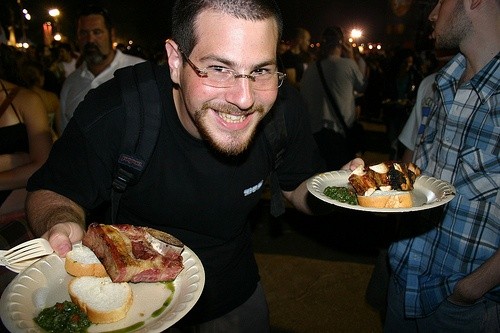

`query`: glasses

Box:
[179,47,285,91]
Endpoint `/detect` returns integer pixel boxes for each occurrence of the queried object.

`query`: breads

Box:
[357,186,414,208]
[68,275,132,324]
[348,161,421,191]
[64,246,112,277]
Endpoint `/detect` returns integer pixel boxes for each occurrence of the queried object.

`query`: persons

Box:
[23,0,366,333]
[0,7,147,293]
[279,24,415,175]
[385,1,500,333]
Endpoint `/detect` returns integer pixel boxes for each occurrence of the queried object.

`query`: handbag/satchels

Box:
[322,131,362,168]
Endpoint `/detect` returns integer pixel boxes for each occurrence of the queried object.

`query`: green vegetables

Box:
[33,300,91,333]
[323,186,359,205]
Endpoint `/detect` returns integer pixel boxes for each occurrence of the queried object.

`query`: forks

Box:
[3,237,54,265]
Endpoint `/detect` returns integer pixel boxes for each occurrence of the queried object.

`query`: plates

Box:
[306,170,456,215]
[0,240,205,333]
